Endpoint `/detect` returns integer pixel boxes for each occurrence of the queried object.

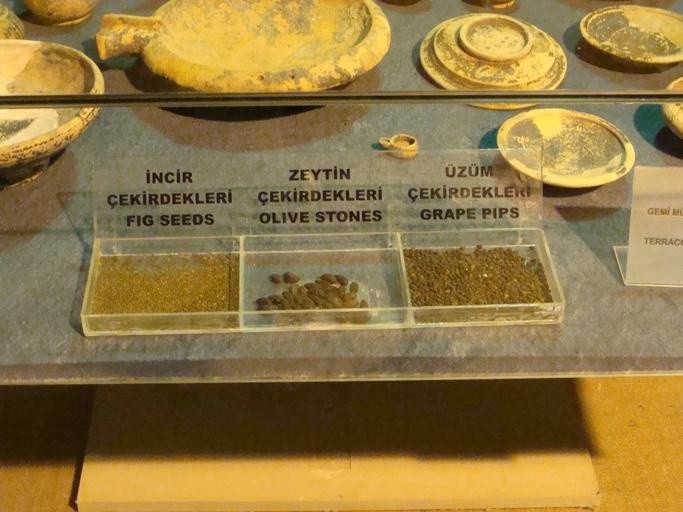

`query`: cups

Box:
[376,132,421,160]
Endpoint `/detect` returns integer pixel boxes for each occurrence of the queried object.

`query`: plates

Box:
[415,12,571,112]
[0,37,103,174]
[96,0,393,100]
[575,4,683,73]
[492,107,638,191]
